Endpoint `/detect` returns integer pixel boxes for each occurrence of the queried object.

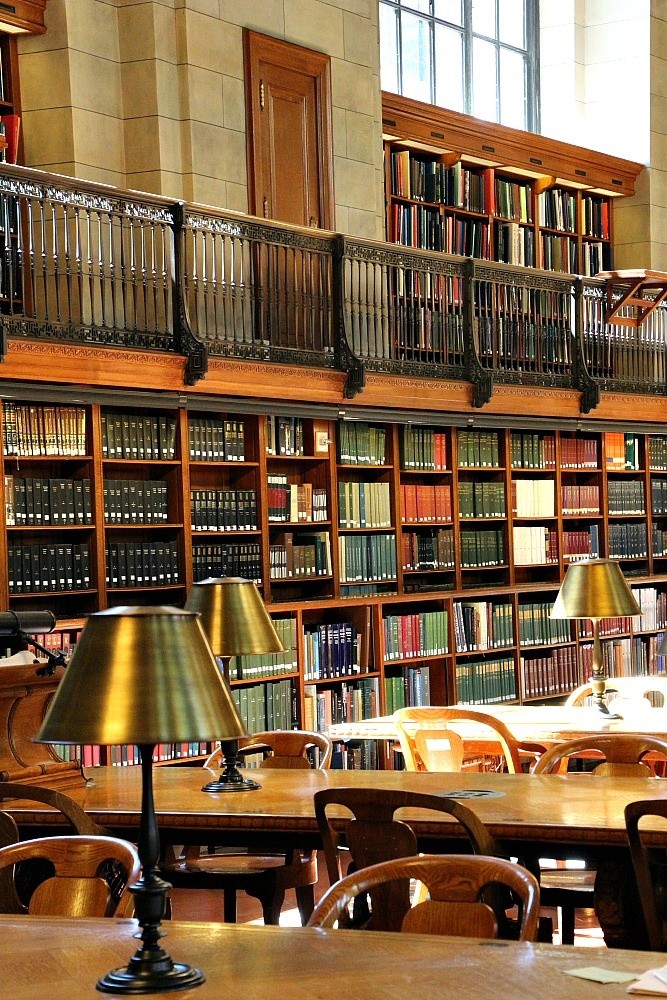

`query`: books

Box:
[26,631,81,660]
[512,434,559,564]
[400,427,455,592]
[110,742,207,766]
[457,430,506,568]
[580,587,666,684]
[384,611,449,771]
[187,420,262,585]
[453,601,515,705]
[519,603,579,699]
[391,150,611,363]
[215,618,299,768]
[1,402,96,591]
[560,437,600,562]
[264,416,332,579]
[101,413,179,585]
[337,422,398,599]
[302,609,380,771]
[604,432,667,559]
[54,744,100,767]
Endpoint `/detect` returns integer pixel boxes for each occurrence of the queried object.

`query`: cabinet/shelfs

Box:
[0,376,667,729]
[382,92,645,376]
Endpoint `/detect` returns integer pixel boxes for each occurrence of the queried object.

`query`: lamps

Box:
[546,557,645,720]
[31,608,251,993]
[176,576,284,792]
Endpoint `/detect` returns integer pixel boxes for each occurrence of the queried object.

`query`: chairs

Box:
[0,676,667,942]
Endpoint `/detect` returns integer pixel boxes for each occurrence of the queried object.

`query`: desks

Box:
[321,704,667,775]
[0,914,667,1000]
[2,765,667,889]
